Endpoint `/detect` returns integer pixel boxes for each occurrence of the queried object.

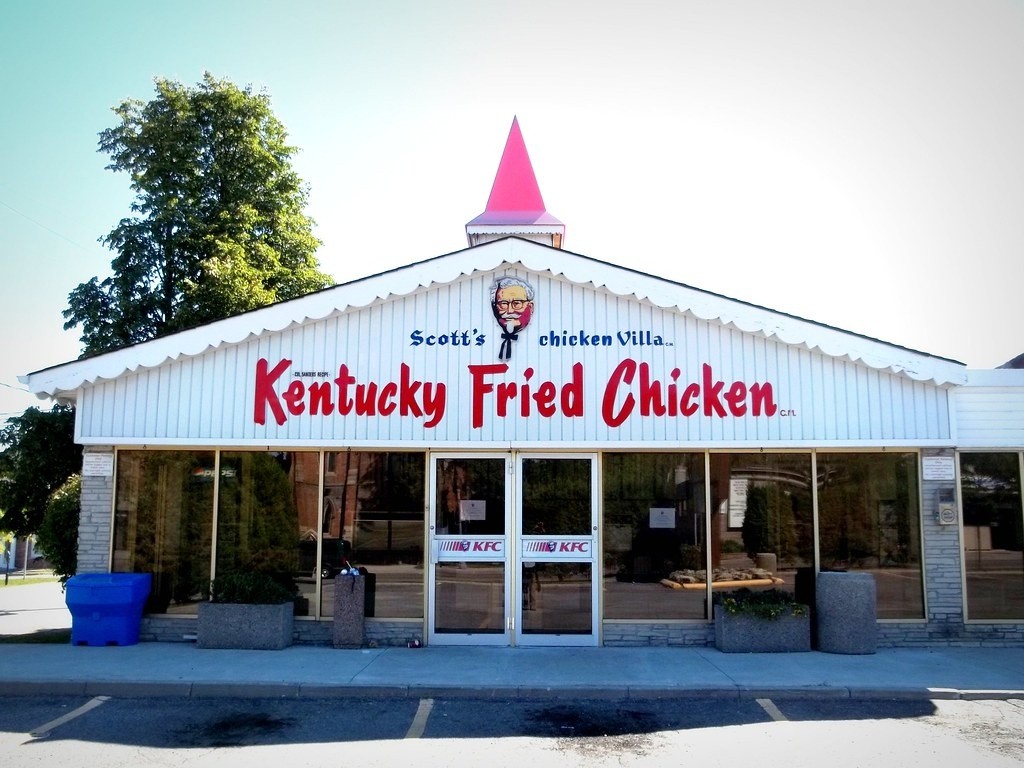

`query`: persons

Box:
[490,278,535,335]
[523,522,545,610]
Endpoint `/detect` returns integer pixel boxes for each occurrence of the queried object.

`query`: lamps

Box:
[674,454,688,485]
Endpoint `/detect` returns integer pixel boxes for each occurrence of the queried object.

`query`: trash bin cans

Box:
[332,575,366,650]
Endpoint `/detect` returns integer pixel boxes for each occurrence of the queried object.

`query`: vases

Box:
[714,606,811,653]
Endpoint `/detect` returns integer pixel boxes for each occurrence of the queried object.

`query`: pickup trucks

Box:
[297,537,352,578]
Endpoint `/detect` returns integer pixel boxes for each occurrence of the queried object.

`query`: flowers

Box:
[719,592,808,620]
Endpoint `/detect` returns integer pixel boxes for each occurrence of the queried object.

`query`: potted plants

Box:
[293,581,310,618]
[197,555,293,649]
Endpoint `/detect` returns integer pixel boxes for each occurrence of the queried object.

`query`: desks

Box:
[661,577,785,599]
